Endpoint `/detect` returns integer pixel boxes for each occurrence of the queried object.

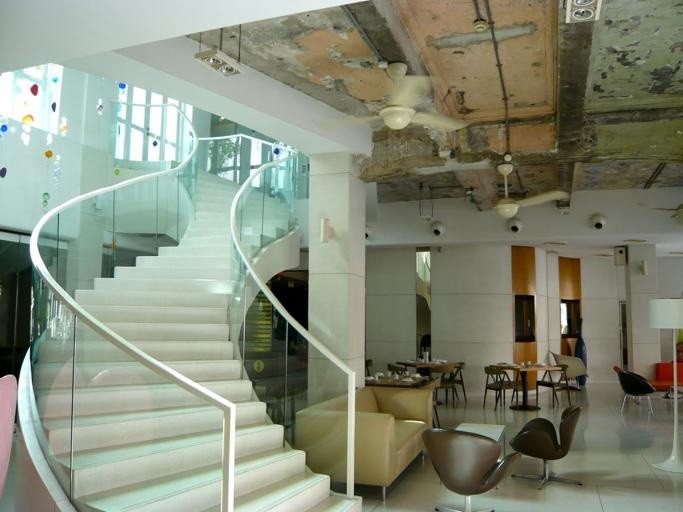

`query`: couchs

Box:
[650,363,683,392]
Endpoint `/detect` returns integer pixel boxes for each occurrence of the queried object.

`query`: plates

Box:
[401,373,420,382]
[408,358,446,365]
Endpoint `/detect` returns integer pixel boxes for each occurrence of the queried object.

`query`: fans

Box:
[494,164,568,220]
[316,62,467,131]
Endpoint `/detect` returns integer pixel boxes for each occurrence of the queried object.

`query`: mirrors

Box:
[560,300,580,339]
[516,295,535,342]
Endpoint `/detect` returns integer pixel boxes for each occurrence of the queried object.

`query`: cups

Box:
[388,370,409,380]
[519,360,532,366]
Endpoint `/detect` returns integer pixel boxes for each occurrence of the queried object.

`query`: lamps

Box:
[366,230,372,240]
[432,223,445,237]
[195,25,244,78]
[508,220,522,233]
[649,299,683,473]
[590,214,606,231]
